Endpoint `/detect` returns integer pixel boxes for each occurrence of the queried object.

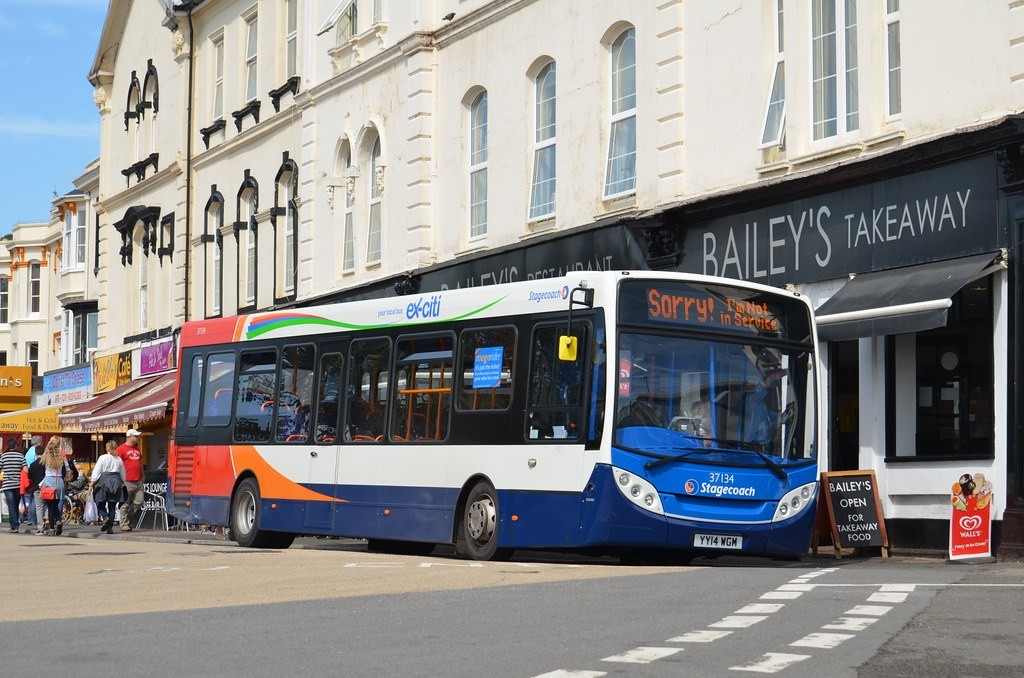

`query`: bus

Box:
[165,271,821,561]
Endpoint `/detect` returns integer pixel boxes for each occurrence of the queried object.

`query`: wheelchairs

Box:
[60,492,103,526]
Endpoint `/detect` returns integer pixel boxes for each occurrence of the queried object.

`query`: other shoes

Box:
[123,525,134,531]
[9,530,19,533]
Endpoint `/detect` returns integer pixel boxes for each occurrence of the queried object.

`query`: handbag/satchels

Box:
[84,501,98,521]
[40,488,56,500]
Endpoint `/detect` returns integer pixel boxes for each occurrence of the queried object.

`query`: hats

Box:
[126,429,141,436]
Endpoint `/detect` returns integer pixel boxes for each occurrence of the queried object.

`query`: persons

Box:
[740,353,793,451]
[89,440,128,534]
[0,439,28,533]
[115,429,145,531]
[24,434,79,537]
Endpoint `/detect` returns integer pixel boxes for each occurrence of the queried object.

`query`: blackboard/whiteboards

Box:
[821,470,888,547]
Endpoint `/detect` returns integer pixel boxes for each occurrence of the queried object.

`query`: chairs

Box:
[134,491,195,532]
[212,387,434,443]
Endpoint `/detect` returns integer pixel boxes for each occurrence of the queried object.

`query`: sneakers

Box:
[101,517,113,534]
[35,520,49,536]
[47,521,63,536]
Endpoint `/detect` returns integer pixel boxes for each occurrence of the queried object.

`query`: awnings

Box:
[811,251,1009,343]
[57,370,177,433]
